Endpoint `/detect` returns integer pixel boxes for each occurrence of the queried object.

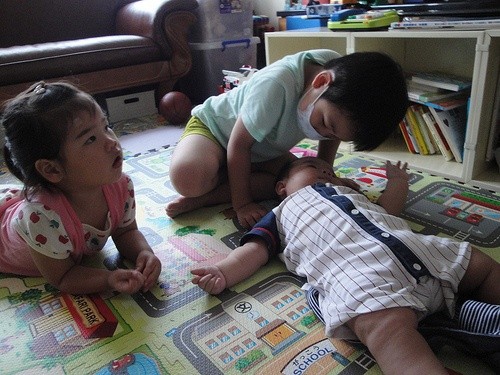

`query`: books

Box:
[399,71,471,163]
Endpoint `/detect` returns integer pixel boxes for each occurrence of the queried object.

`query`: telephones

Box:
[327,8,398,31]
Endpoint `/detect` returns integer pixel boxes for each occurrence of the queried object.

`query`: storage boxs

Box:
[182,37,261,97]
[105,90,158,124]
[198,0,254,42]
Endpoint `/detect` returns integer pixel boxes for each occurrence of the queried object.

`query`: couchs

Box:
[0,0,200,115]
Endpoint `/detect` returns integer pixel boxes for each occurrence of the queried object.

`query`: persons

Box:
[0,80,161,295]
[166,49,408,229]
[190,157,500,375]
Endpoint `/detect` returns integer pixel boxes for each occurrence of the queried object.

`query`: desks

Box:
[265,27,500,193]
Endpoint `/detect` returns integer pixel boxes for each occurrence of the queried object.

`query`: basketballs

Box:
[160,91,192,123]
